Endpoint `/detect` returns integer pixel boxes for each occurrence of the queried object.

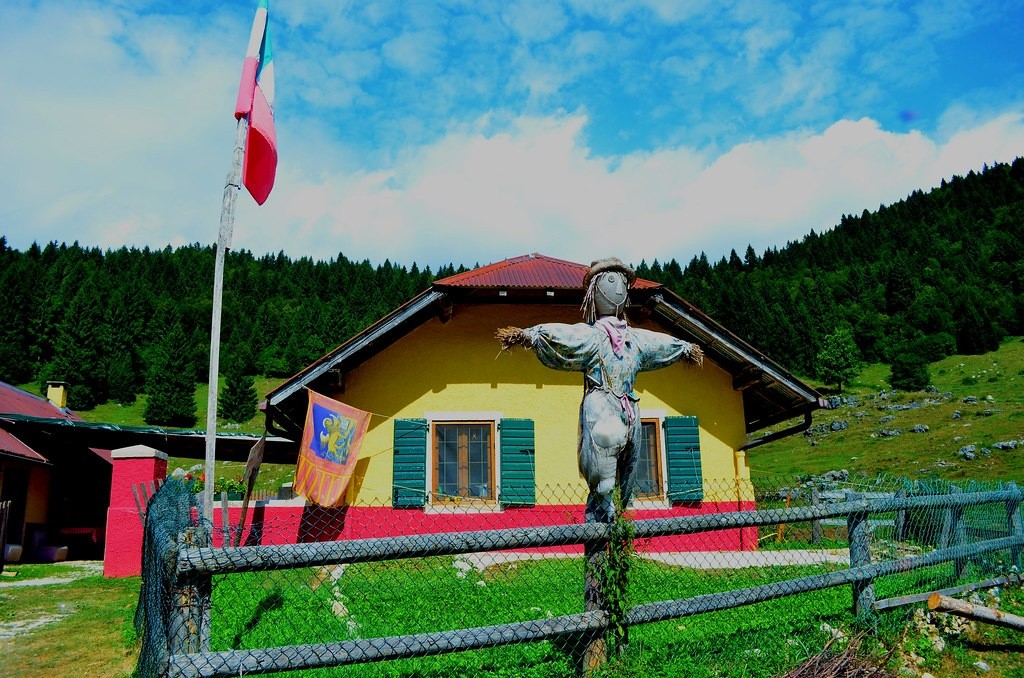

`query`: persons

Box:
[497,257,703,523]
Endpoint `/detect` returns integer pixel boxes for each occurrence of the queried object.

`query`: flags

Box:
[234,0,278,207]
[293,390,372,507]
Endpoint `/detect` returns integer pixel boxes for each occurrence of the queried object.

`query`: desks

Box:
[59,527,105,553]
